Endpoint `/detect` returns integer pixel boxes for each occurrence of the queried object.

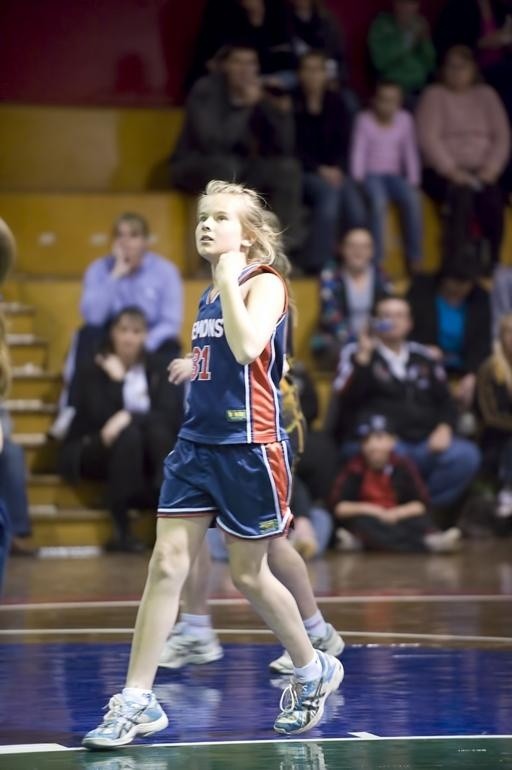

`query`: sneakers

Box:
[267,620,348,671]
[154,631,226,669]
[81,686,169,750]
[272,649,344,735]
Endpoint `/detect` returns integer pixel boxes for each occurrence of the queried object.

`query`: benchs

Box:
[2,101,512,565]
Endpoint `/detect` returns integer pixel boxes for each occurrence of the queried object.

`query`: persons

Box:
[287,228,511,557]
[158,213,344,673]
[54,211,184,553]
[172,0,512,279]
[0,209,33,585]
[83,181,345,749]
